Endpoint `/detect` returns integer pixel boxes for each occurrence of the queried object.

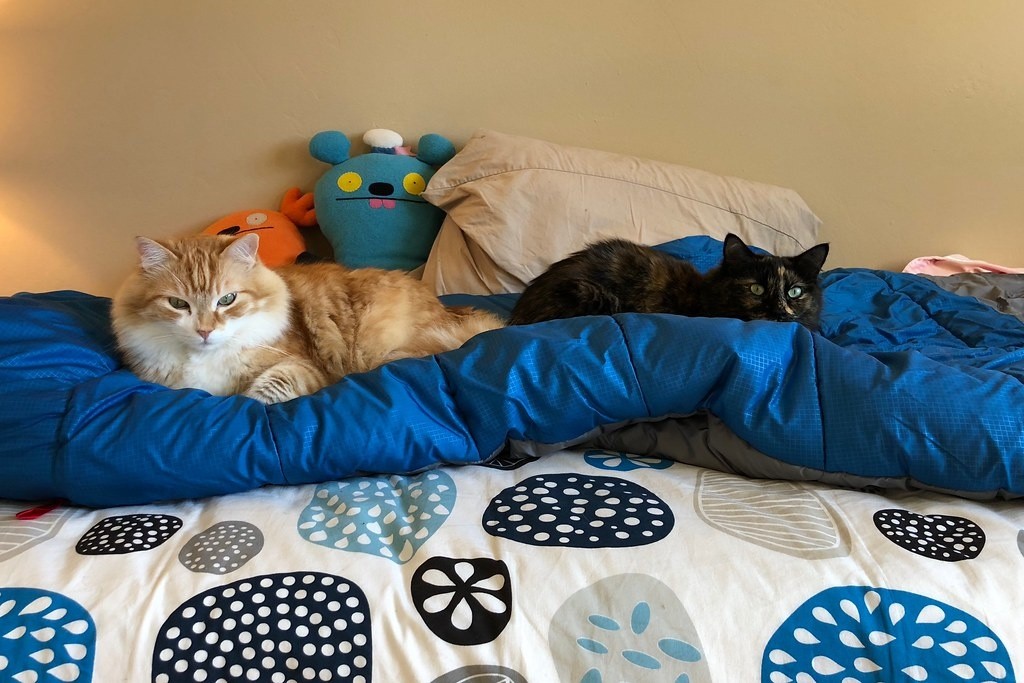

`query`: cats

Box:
[111,232,509,403]
[507,233,830,331]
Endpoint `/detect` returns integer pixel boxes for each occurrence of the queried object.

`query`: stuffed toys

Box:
[201,188,317,273]
[309,128,456,271]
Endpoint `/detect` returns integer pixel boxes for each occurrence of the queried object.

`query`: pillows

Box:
[421,133,825,297]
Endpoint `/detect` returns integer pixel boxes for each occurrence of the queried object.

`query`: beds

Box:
[0,269,1023,683]
